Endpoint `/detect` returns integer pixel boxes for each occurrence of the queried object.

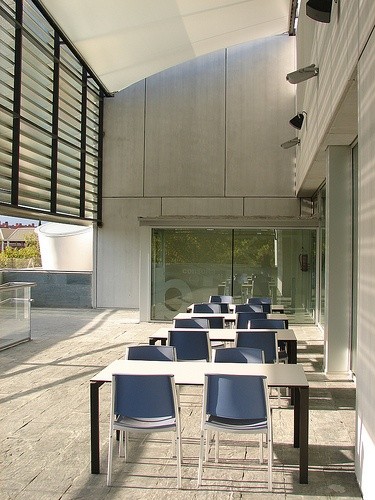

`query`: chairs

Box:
[107,374,182,489]
[249,318,289,396]
[191,295,273,329]
[197,373,272,490]
[119,345,177,456]
[168,330,210,361]
[174,319,210,328]
[211,349,266,464]
[234,331,280,409]
[226,272,270,297]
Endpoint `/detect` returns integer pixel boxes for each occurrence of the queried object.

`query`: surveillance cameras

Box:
[280,137,300,149]
[286,64,318,83]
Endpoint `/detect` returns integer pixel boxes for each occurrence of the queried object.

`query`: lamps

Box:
[281,137,300,149]
[287,64,318,84]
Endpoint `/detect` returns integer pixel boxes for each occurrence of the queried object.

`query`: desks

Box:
[91,359,309,485]
[149,327,298,405]
[219,276,277,294]
[174,313,289,356]
[188,303,284,313]
